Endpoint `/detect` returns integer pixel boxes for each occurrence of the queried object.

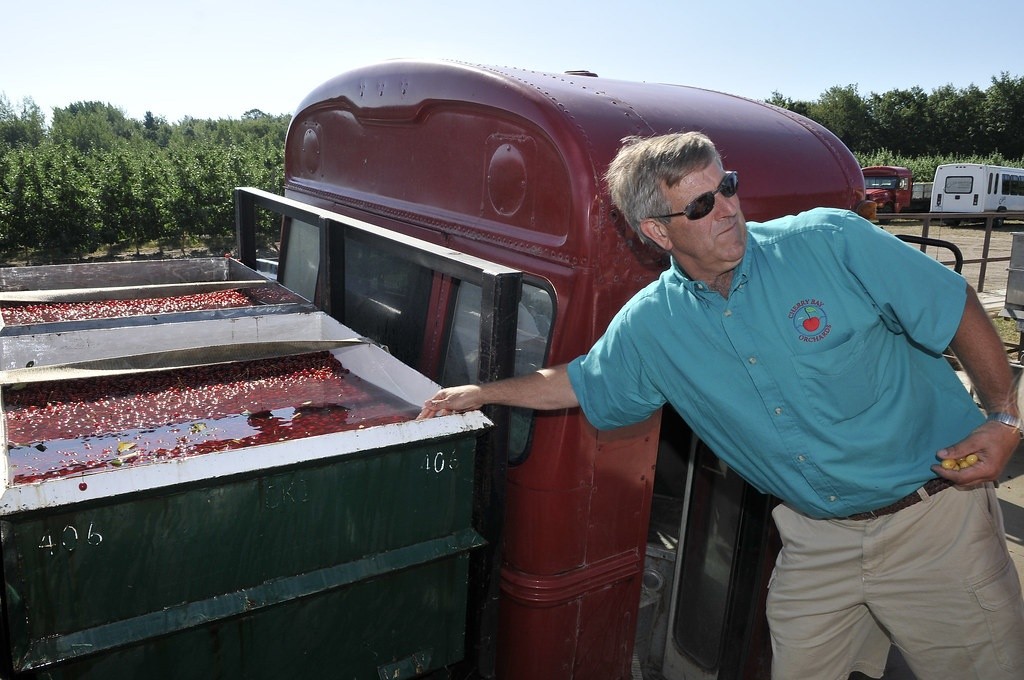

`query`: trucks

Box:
[928,164,1024,227]
[1,60,868,680]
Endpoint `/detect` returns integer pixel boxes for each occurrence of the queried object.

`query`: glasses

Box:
[649,170,739,220]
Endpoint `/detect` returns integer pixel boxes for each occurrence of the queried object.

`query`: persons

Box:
[417,130,1024,680]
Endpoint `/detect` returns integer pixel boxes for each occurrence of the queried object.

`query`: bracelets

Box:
[985,412,1024,439]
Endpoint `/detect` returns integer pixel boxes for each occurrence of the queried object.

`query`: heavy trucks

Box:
[862,166,931,220]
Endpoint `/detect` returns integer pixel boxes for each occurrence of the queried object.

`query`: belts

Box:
[838,477,955,521]
